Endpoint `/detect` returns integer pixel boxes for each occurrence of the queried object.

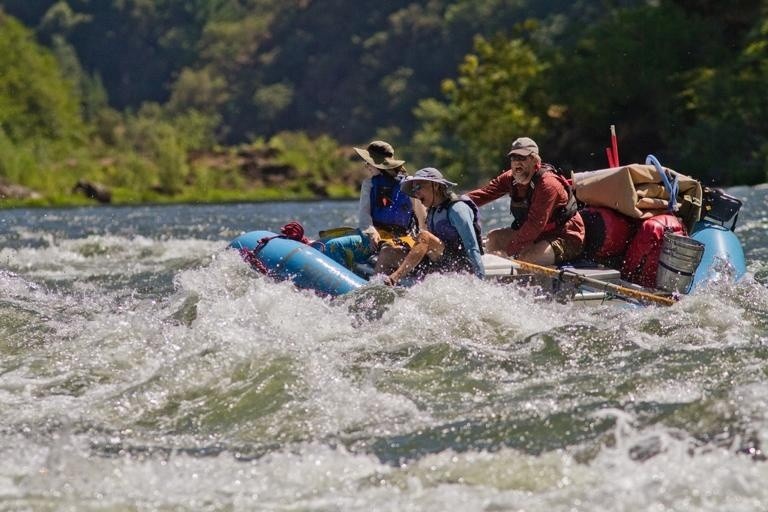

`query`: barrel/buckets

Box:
[687,226,746,291]
[657,233,705,292]
[225,228,365,299]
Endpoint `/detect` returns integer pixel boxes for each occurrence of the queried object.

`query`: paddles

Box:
[319,227,357,238]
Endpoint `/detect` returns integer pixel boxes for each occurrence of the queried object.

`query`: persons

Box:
[321,141,429,271]
[463,137,587,265]
[385,167,486,287]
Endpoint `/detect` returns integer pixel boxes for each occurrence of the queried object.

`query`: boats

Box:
[224,217,746,324]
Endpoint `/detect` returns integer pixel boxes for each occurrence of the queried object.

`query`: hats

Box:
[508,137,538,156]
[400,167,458,196]
[354,141,405,170]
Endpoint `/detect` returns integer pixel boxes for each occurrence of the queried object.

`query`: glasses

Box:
[410,184,421,194]
[510,155,527,161]
